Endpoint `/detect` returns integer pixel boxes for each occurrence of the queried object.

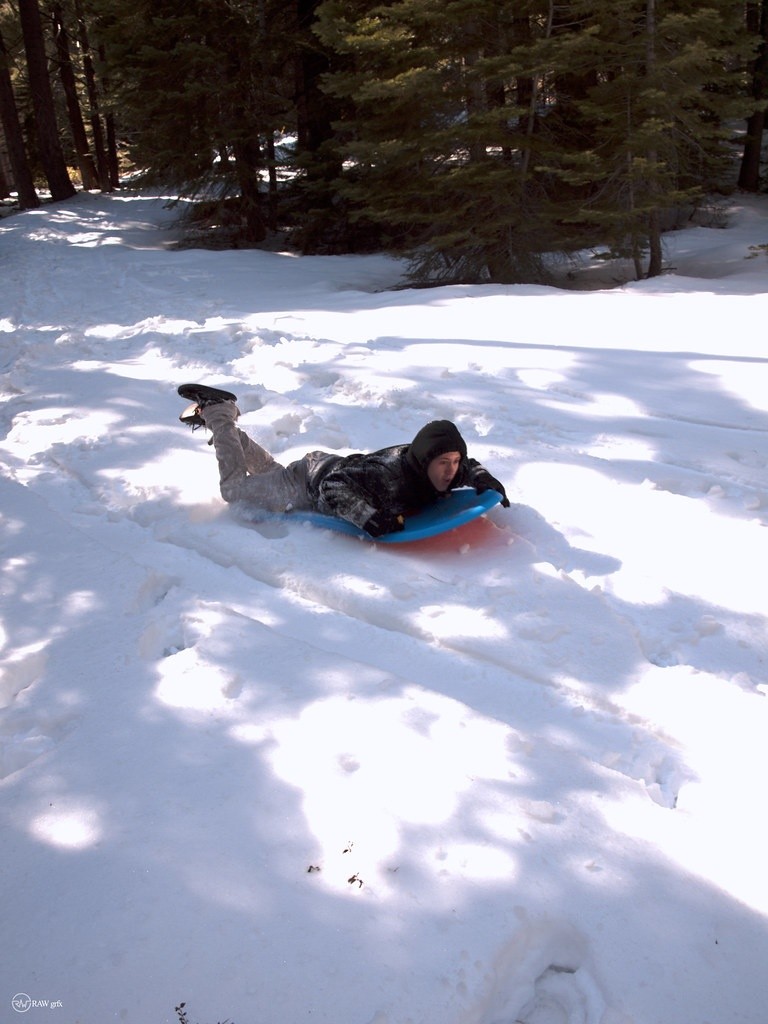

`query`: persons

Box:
[178,384,509,537]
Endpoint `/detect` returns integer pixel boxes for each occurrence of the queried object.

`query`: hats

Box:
[409,420,467,499]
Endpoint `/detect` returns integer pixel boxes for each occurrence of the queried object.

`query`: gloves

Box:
[363,508,405,538]
[475,472,510,508]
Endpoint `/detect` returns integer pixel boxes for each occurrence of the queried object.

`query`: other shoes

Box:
[177,384,237,430]
